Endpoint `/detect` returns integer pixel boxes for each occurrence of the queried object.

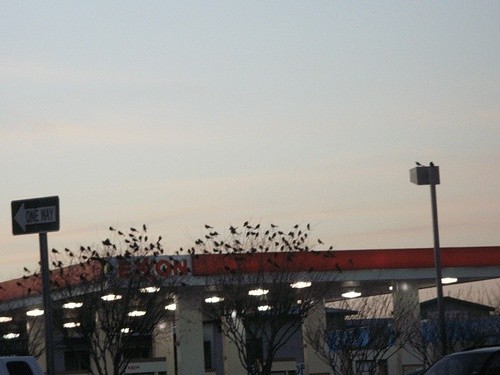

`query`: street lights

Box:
[410,161,450,356]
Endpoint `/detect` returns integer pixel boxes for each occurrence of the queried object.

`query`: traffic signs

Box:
[11,196,60,235]
